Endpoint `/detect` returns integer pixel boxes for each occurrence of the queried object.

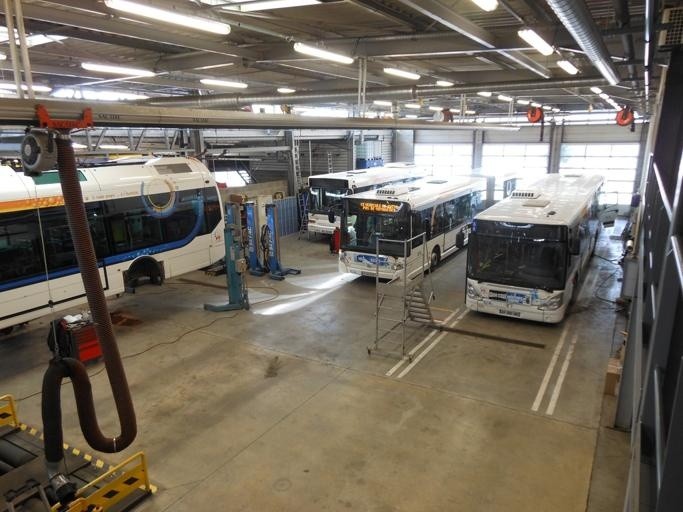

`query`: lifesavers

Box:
[527,107,542,123]
[616,108,634,125]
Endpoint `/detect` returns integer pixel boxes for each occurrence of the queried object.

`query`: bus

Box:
[0,152,226,330]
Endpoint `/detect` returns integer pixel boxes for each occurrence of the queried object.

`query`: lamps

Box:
[199,78,249,89]
[81,61,157,78]
[477,91,561,113]
[589,85,624,112]
[102,0,232,36]
[517,26,580,76]
[292,41,354,67]
[384,67,455,87]
